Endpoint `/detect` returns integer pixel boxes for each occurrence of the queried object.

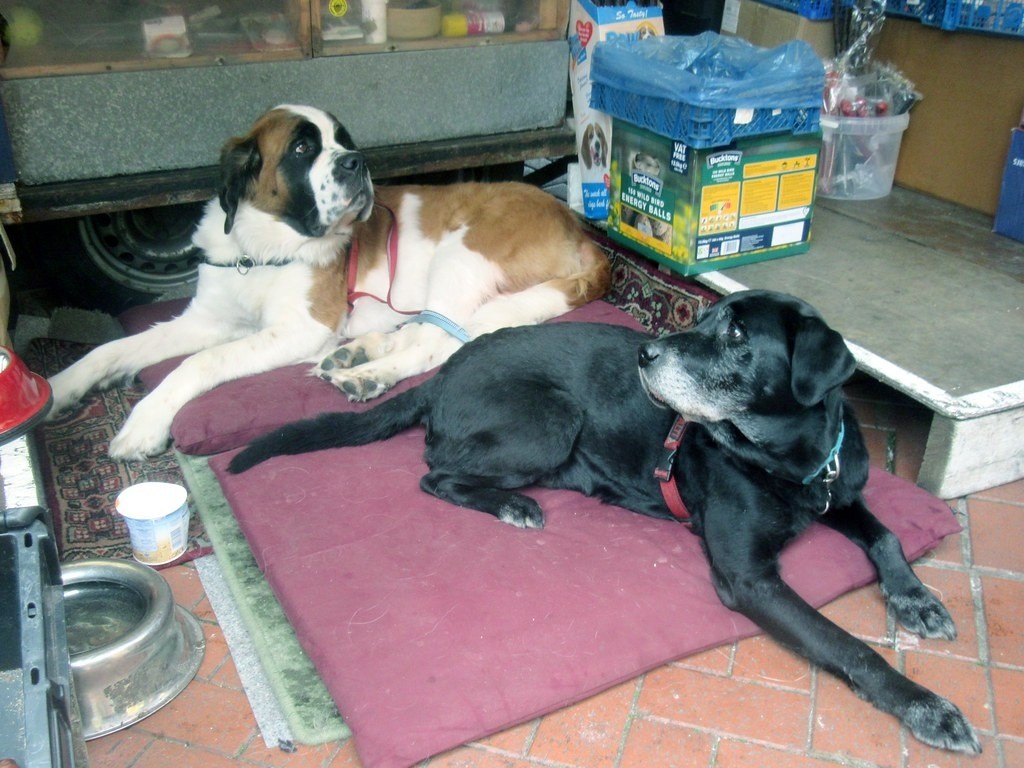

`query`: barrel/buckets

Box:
[815,109,910,200]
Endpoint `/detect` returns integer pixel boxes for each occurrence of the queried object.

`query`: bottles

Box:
[441,11,505,36]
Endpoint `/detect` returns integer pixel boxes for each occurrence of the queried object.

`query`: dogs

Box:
[30,102,984,758]
[580,123,607,170]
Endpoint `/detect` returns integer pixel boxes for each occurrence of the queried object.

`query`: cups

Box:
[115,481,190,566]
[361,0,387,45]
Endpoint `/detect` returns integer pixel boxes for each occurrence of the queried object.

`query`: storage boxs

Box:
[567,0,666,218]
[606,116,822,277]
[718,0,836,59]
[992,124,1024,243]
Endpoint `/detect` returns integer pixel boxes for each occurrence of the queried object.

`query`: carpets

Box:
[23,225,726,571]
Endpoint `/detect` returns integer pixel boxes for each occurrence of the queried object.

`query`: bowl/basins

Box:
[59,557,206,742]
[386,3,442,39]
[0,343,53,447]
